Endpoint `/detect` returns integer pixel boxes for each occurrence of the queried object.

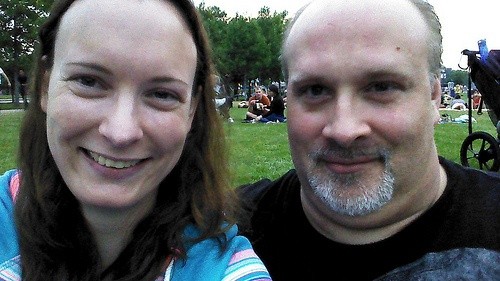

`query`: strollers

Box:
[460,47,500,173]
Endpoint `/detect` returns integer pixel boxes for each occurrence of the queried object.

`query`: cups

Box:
[256,103,260,109]
[478,40,489,62]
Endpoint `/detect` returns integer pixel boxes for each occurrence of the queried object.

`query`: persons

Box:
[0,68,11,87]
[210,73,482,124]
[221,0,500,281]
[15,69,29,110]
[0,0,271,281]
